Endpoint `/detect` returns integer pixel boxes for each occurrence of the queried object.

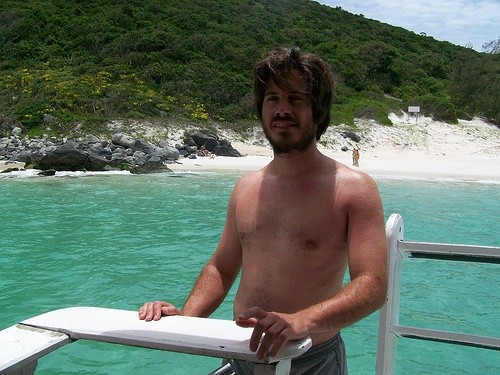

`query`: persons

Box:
[352,148,356,165]
[137,45,389,375]
[201,145,208,156]
[354,150,359,167]
